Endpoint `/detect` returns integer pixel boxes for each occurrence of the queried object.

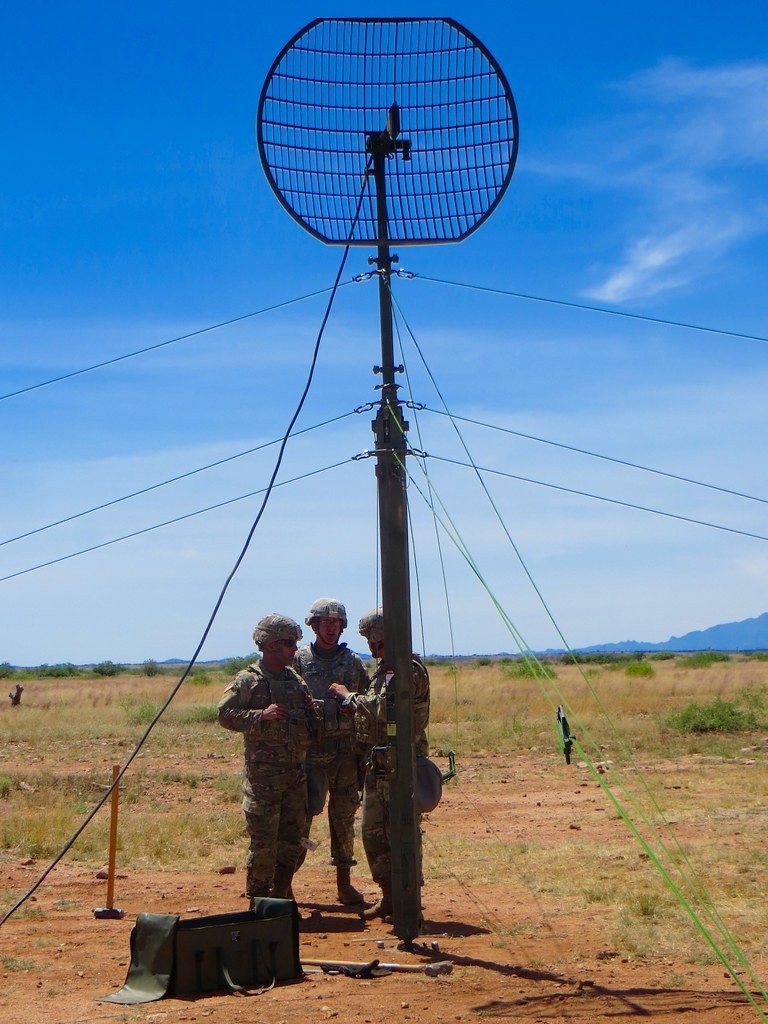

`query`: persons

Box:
[289,598,371,903]
[218,612,324,911]
[329,606,430,923]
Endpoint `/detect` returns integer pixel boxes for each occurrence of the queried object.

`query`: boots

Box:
[337,867,363,904]
[385,887,424,923]
[359,884,393,919]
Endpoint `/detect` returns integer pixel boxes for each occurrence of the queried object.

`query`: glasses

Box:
[317,619,342,627]
[276,639,297,648]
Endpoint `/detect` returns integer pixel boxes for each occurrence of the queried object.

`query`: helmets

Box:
[306,601,347,628]
[359,613,384,635]
[254,617,303,647]
